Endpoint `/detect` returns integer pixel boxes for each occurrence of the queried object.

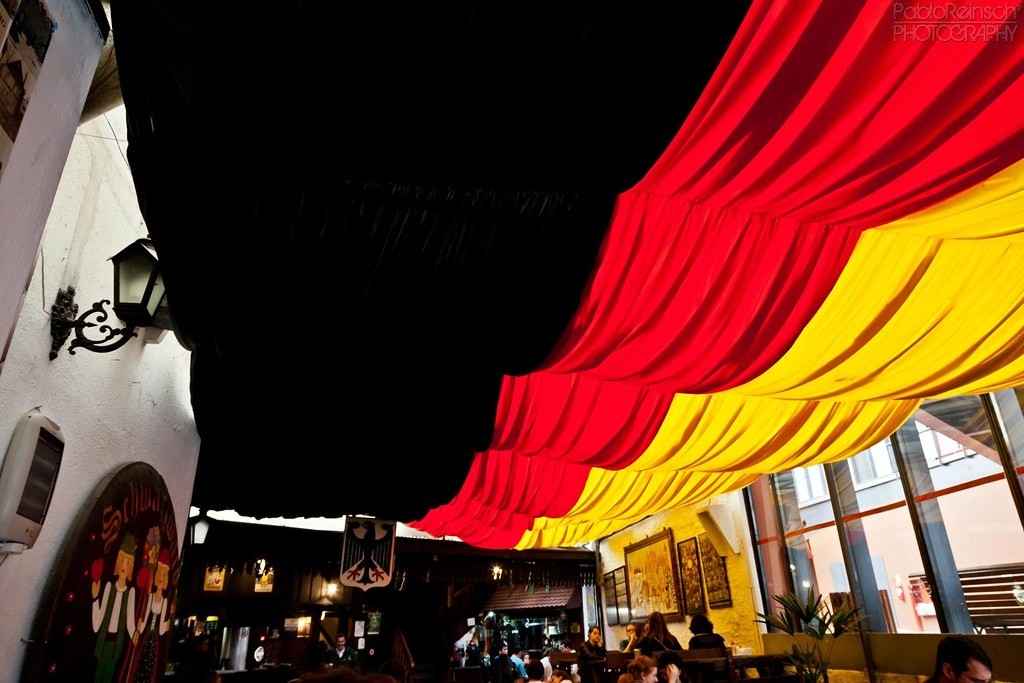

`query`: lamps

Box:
[49,234,166,360]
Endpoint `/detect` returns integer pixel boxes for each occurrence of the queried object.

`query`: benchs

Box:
[652,647,732,661]
[606,652,635,683]
[550,653,578,675]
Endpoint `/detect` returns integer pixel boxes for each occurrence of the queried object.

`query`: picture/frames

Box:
[624,527,683,624]
[603,571,619,626]
[677,537,706,615]
[614,565,629,626]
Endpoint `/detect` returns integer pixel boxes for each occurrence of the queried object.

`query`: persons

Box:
[174,635,222,683]
[458,612,728,683]
[325,634,357,668]
[924,635,992,683]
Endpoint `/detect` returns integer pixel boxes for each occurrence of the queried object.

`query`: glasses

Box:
[961,671,997,683]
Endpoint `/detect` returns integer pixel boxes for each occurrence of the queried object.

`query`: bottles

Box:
[730,640,737,656]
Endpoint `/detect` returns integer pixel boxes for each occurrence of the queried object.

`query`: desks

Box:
[590,660,606,683]
[559,659,578,663]
[682,653,813,683]
